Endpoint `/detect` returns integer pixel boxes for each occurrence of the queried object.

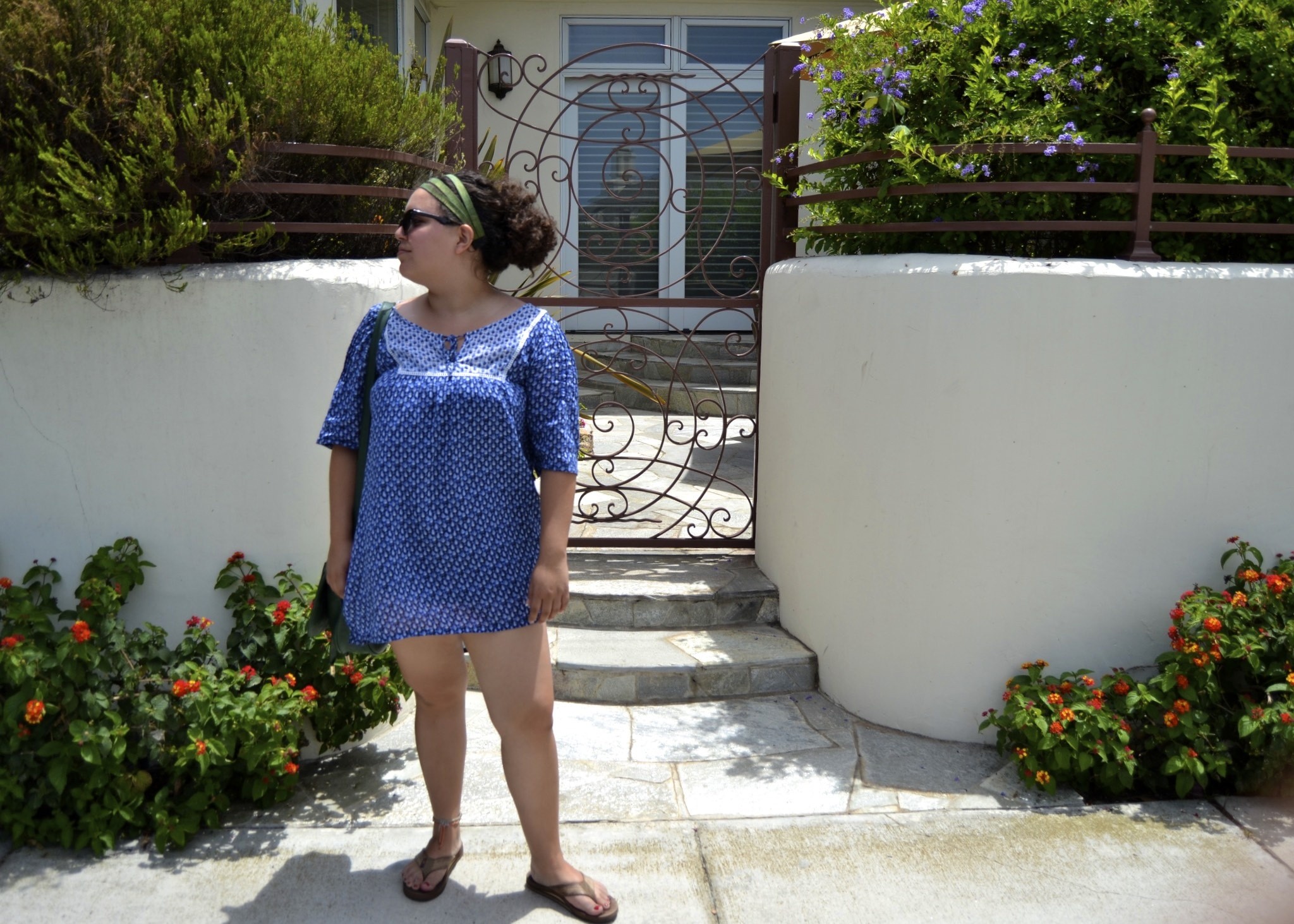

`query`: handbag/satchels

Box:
[304,561,384,661]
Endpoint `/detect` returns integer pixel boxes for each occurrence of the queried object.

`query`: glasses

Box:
[397,210,478,249]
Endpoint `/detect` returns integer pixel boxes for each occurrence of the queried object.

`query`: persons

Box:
[316,169,621,924]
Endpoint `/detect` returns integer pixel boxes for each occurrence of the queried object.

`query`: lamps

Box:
[486,38,513,99]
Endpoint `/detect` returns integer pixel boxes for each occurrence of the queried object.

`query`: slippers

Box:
[403,835,466,903]
[525,862,618,923]
